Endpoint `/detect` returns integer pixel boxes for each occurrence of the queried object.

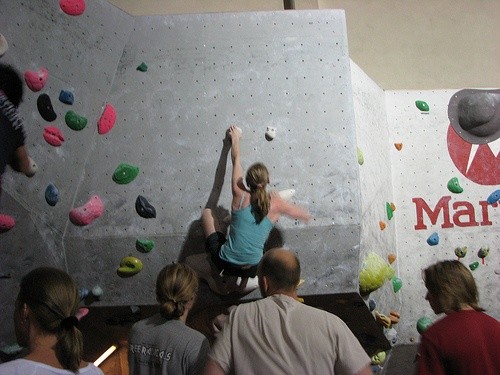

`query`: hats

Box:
[448,89,500,144]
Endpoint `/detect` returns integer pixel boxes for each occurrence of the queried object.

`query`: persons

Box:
[128,262,209,375]
[202,125,309,289]
[0,267,104,375]
[201,249,372,375]
[416,260,500,374]
